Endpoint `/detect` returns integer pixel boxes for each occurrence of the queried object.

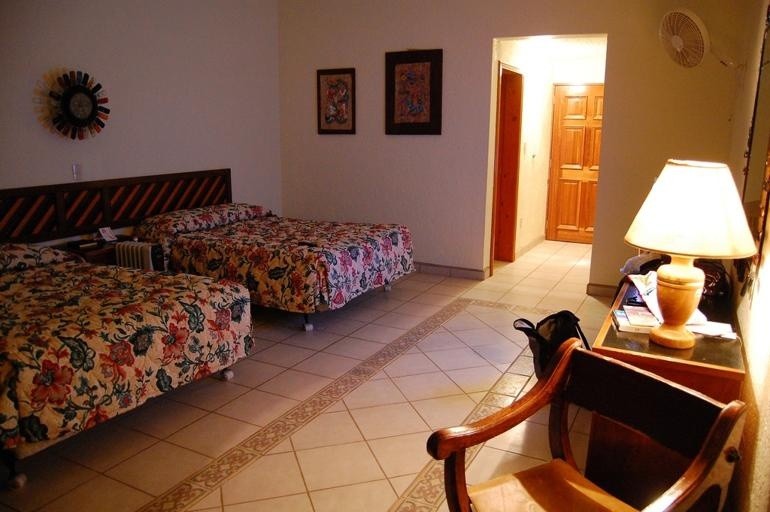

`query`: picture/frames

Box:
[385,47,443,135]
[317,67,355,135]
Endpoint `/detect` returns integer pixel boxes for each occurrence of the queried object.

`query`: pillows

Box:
[1,244,83,273]
[138,203,271,239]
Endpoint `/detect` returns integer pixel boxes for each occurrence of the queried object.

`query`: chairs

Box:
[427,336,748,512]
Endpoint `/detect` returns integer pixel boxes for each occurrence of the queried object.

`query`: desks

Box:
[585,278,746,512]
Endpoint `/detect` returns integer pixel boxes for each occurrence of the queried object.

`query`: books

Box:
[623,305,657,327]
[611,309,657,334]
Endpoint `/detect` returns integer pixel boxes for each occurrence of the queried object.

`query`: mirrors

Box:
[726,5,770,292]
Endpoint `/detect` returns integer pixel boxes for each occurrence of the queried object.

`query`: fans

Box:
[659,9,743,72]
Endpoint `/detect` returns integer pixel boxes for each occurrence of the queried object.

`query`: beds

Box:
[0,243,256,488]
[134,204,415,332]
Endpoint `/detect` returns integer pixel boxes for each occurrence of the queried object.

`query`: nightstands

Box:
[52,235,136,266]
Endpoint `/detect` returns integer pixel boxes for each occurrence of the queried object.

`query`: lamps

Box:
[623,157,757,348]
